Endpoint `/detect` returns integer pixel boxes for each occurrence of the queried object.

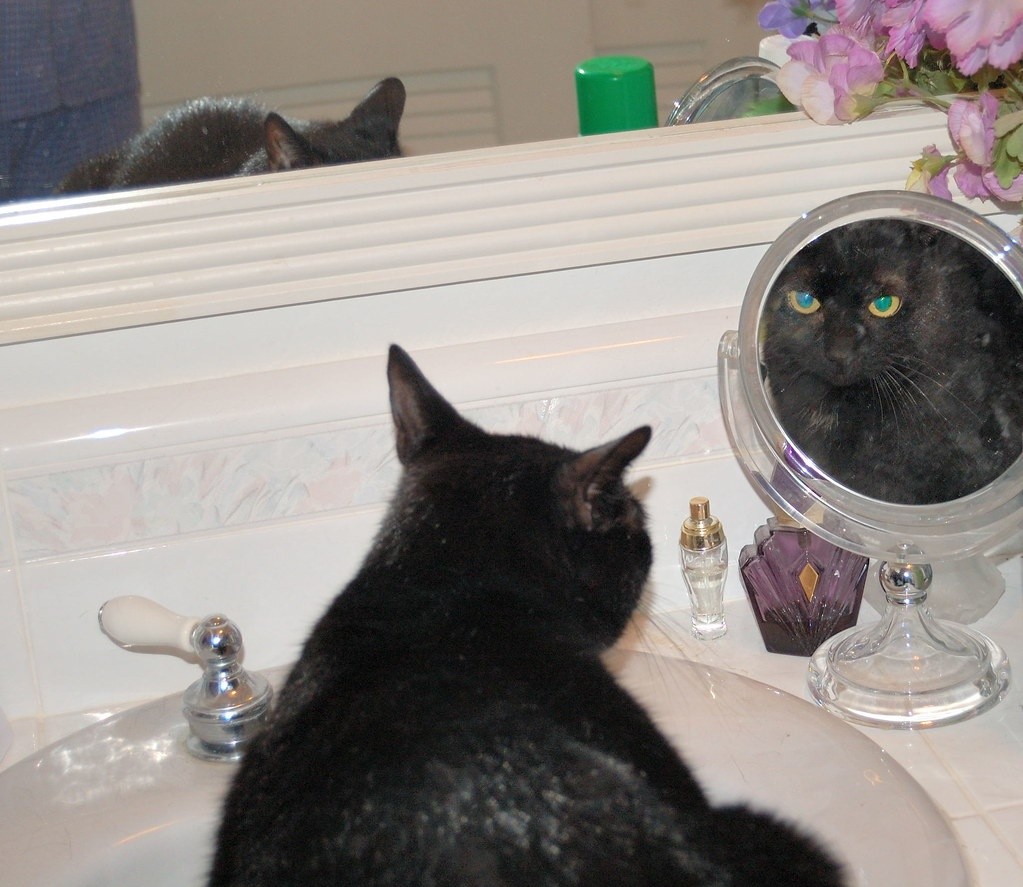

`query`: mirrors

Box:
[0,0,796,208]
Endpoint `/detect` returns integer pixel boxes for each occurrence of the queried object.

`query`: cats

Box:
[204,343,851,887]
[52,75,407,198]
[762,215,1023,506]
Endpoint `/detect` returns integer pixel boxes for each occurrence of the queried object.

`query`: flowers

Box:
[759,0,1023,212]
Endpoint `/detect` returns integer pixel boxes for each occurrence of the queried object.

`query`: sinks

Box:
[0,646,968,887]
